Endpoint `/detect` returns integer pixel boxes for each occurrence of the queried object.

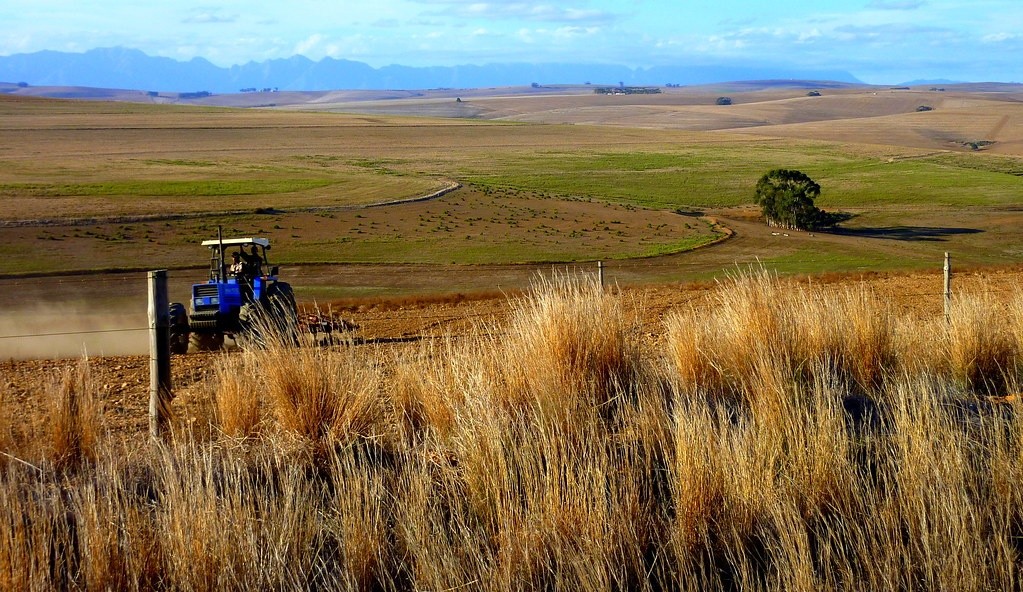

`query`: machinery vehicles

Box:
[169,225,298,355]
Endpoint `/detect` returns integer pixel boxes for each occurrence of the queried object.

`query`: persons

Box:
[229,246,264,277]
